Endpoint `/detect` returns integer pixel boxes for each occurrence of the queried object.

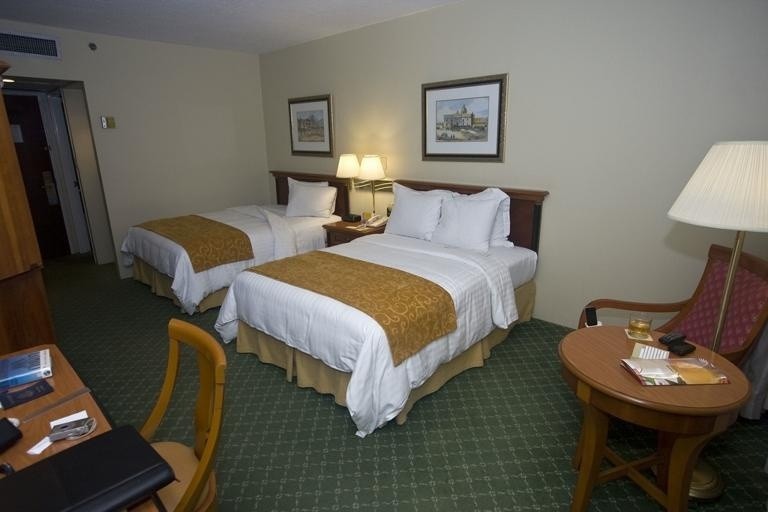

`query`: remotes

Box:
[658,331,687,345]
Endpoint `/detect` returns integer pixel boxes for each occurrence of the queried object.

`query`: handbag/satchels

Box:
[0,424,175,512]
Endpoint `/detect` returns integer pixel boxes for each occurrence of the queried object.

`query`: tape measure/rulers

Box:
[22,386,92,424]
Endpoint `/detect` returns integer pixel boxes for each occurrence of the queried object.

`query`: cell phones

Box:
[667,342,696,357]
[585,307,597,326]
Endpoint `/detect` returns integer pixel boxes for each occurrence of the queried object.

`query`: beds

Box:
[214,180,549,437]
[120,171,352,314]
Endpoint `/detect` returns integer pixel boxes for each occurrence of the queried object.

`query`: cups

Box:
[627,311,652,340]
[361,208,373,226]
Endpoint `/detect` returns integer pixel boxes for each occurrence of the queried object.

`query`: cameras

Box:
[49,417,91,441]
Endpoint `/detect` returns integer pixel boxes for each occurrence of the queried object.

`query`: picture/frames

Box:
[288,94,335,158]
[422,74,508,163]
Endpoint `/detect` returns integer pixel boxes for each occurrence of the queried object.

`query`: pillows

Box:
[431,196,508,250]
[385,181,442,240]
[287,183,338,218]
[284,177,328,200]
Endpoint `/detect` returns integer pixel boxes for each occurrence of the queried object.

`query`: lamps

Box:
[336,154,371,192]
[359,154,392,193]
[668,141,768,500]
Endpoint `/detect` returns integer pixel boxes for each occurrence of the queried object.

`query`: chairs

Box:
[578,245,767,364]
[126,318,225,512]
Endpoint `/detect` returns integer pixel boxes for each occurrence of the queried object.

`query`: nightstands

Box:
[322,214,384,246]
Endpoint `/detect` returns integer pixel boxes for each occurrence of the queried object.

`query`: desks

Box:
[1,344,165,512]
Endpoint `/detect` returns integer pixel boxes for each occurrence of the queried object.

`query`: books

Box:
[620,358,729,385]
[0,349,53,389]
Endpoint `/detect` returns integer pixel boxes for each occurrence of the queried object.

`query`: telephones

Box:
[365,214,389,228]
